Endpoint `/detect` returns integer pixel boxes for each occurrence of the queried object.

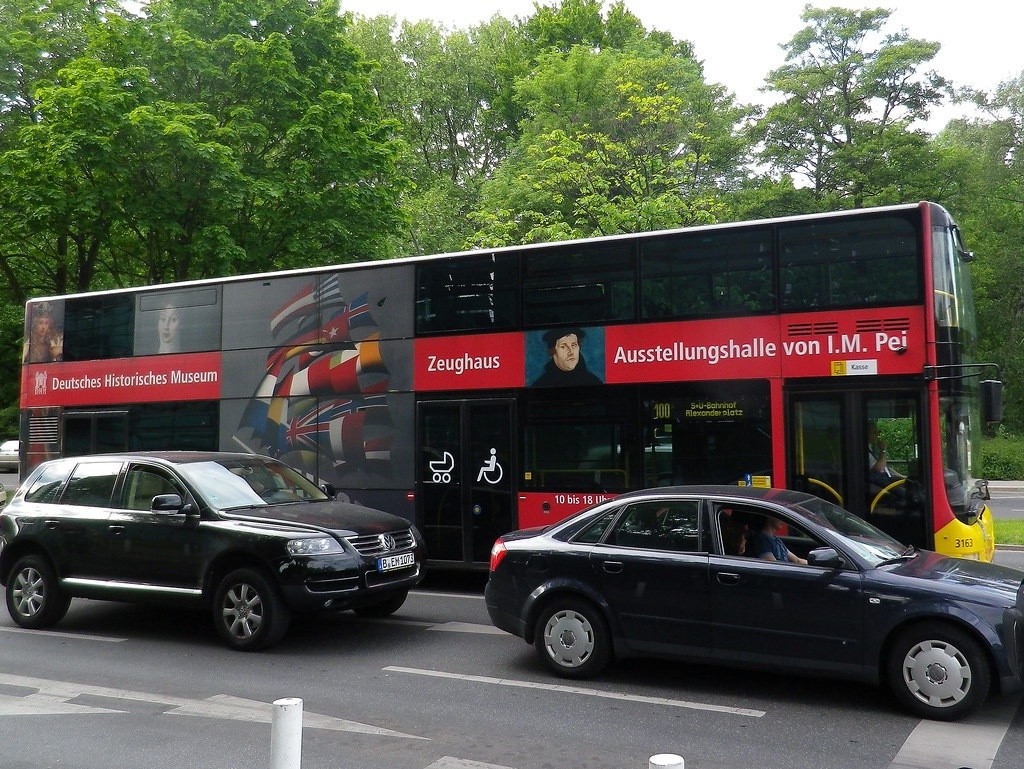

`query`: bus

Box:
[18,200,997,574]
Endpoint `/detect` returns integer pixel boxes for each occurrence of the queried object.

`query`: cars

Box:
[0,440,19,473]
[484,484,1024,722]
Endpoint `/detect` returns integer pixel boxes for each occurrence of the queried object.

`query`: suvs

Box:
[0,451,428,651]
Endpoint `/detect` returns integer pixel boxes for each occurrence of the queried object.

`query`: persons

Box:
[752,514,808,565]
[724,522,748,556]
[866,420,887,499]
[244,474,264,493]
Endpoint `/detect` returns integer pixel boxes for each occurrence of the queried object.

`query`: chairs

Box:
[134,475,164,508]
[719,510,748,529]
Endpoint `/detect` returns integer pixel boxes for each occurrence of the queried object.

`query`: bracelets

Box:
[881,450,886,452]
